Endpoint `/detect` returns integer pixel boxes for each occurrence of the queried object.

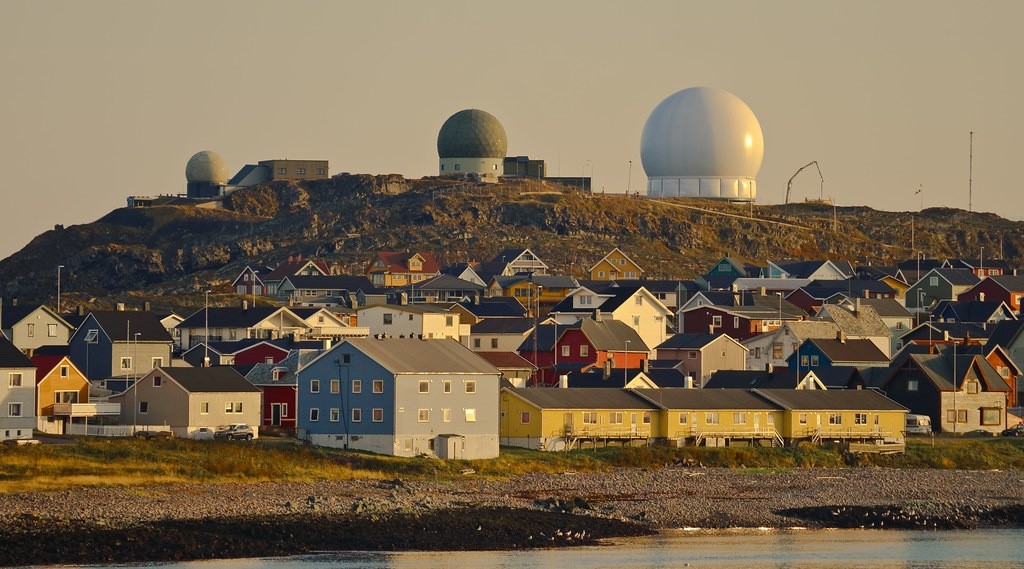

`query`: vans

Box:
[905,413,932,436]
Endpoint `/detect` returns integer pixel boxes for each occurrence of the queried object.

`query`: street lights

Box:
[57,265,65,314]
[918,251,922,281]
[981,246,984,277]
[797,339,803,386]
[134,333,141,437]
[624,341,630,387]
[537,285,542,319]
[929,315,937,347]
[554,311,561,366]
[205,290,213,358]
[953,341,960,434]
[917,288,923,327]
[528,282,533,311]
[253,271,259,309]
[776,292,782,327]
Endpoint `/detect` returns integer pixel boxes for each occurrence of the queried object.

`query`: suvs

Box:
[214,424,255,441]
